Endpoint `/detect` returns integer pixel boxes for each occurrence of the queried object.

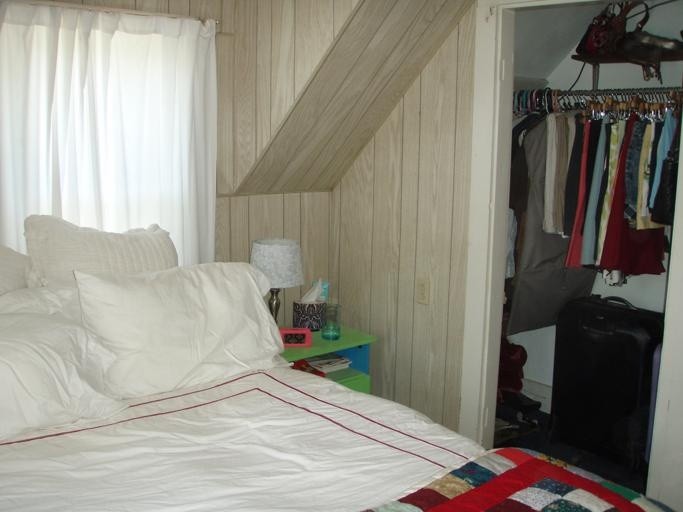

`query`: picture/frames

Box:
[280,328,311,347]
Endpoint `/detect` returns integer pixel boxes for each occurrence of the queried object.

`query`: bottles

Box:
[322,304,339,340]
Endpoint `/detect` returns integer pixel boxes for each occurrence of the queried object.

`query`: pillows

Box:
[68,261,286,400]
[24,215,178,289]
[1,317,124,437]
[0,246,31,287]
[0,285,78,323]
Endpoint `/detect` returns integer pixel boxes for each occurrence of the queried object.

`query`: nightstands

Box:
[279,326,378,396]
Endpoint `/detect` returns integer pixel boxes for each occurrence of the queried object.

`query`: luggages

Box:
[550,294,665,473]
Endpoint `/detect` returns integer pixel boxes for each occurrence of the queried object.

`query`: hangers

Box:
[510,86,683,129]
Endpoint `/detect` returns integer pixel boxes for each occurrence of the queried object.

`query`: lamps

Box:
[252,238,306,324]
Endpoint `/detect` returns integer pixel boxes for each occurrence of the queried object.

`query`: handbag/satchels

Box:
[574,0,649,56]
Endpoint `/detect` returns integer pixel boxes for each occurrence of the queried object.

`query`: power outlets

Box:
[415,279,429,303]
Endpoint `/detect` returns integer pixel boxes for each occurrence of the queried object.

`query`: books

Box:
[305,356,350,373]
[293,359,325,378]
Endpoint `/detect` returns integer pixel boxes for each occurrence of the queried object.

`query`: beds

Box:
[0,365,672,512]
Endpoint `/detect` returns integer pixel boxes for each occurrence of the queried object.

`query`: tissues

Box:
[292,276,326,332]
